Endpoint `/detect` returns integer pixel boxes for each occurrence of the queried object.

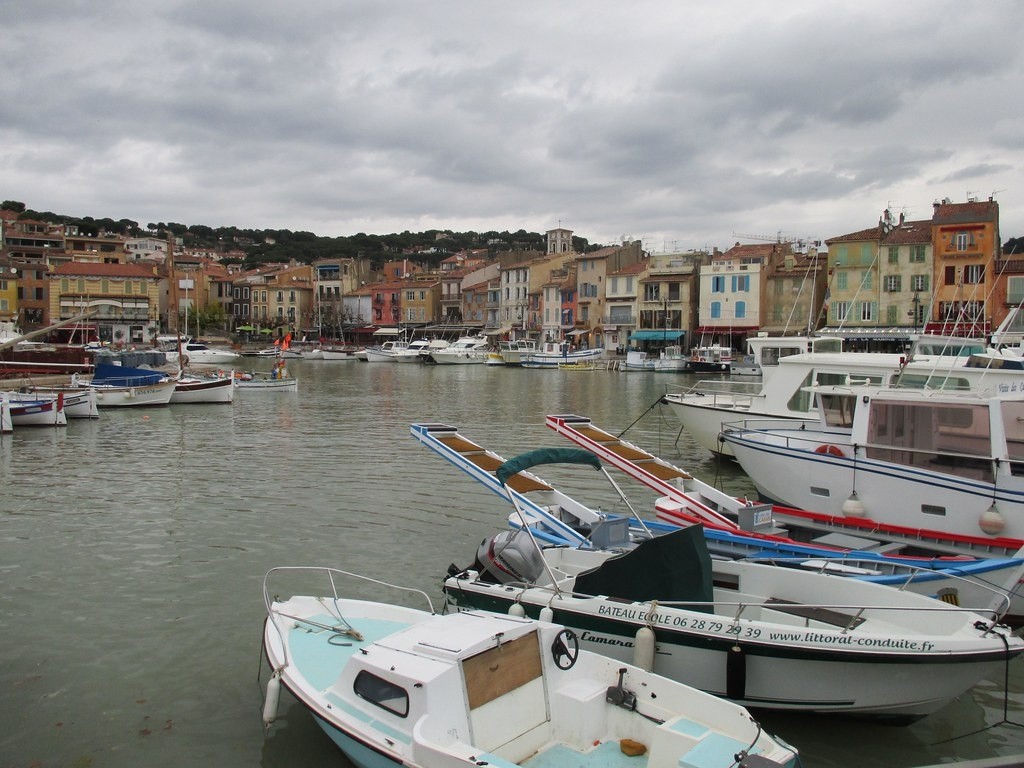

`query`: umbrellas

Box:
[259,328,274,333]
[238,326,256,332]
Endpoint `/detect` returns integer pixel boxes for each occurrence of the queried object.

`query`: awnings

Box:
[566,329,590,336]
[485,327,511,335]
[628,331,685,341]
[815,327,915,338]
[373,328,407,336]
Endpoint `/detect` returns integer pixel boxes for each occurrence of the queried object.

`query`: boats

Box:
[2,298,1024,615]
[411,423,1023,727]
[262,572,797,765]
